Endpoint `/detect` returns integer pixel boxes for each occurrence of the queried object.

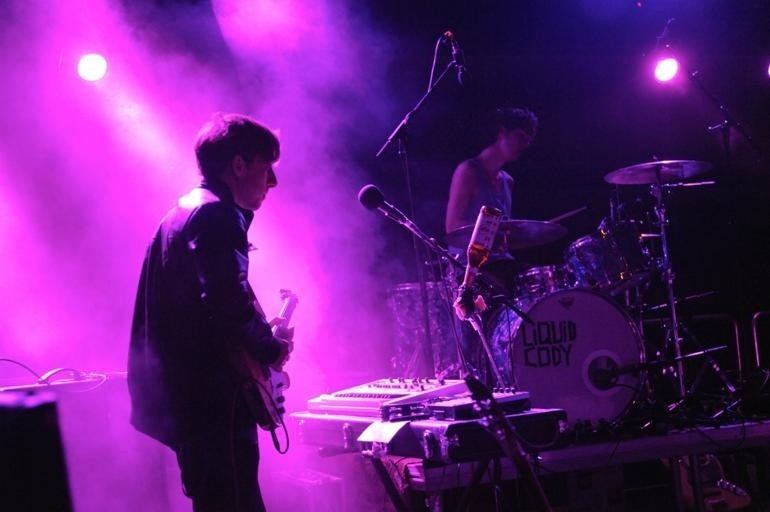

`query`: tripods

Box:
[637,224,739,432]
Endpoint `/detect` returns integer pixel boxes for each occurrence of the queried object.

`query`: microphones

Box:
[450,38,474,87]
[357,183,423,240]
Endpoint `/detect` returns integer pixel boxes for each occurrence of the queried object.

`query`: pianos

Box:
[288,377,484,452]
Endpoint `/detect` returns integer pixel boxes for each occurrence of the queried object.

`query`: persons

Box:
[126,113,294,511]
[445,106,538,369]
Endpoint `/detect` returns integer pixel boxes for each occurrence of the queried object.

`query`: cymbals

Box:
[442,220,563,253]
[604,159,715,185]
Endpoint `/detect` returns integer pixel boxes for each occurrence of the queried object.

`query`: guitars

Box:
[228,289,299,432]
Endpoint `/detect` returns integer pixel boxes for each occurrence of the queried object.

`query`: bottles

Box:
[460,205,504,289]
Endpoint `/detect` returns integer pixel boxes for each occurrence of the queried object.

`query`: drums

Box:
[386,282,461,378]
[511,265,571,299]
[475,285,646,436]
[565,219,657,297]
